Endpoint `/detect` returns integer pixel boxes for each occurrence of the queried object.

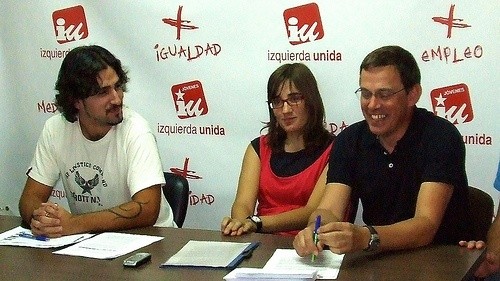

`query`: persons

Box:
[18,44,178,238]
[293,45,476,258]
[221,63,336,235]
[459,159,500,278]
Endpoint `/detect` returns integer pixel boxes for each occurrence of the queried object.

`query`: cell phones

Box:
[123,253,152,267]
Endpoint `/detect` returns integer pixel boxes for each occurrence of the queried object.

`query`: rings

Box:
[45,213,50,216]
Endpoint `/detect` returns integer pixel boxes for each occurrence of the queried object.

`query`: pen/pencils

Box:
[244,249,253,259]
[17,232,50,242]
[312,215,321,263]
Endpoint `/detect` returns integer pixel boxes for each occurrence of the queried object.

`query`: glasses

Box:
[269,96,303,109]
[354,86,406,102]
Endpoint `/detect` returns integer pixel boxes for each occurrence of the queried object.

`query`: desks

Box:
[0,215,487,281]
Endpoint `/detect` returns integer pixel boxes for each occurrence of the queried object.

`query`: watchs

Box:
[246,215,262,233]
[363,225,380,251]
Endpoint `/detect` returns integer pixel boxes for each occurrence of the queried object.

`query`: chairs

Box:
[161,172,189,228]
[438,186,494,242]
